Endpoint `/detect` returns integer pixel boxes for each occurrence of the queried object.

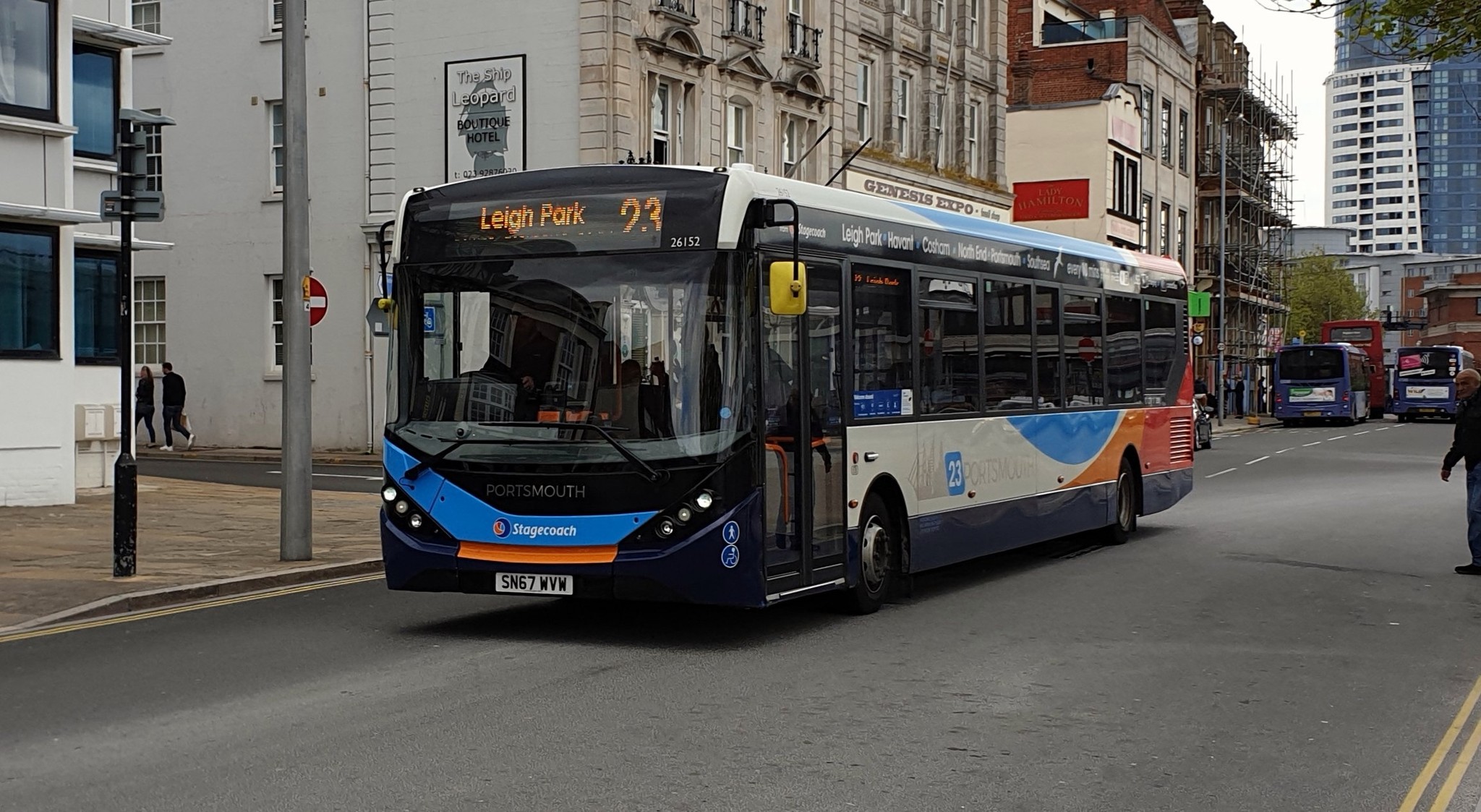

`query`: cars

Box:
[1192,396,1215,451]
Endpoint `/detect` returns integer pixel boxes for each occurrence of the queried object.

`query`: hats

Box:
[1237,376,1242,379]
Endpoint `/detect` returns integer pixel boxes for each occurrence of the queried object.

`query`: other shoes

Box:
[160,445,173,452]
[187,434,196,450]
[1455,564,1481,575]
[144,443,156,449]
[1235,416,1243,419]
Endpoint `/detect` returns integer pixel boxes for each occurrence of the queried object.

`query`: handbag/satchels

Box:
[170,413,186,432]
[185,416,192,432]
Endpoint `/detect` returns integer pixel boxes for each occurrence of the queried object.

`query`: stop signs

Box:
[308,275,329,327]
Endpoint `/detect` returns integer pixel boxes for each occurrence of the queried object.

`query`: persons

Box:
[767,382,832,548]
[920,329,940,386]
[135,366,157,448]
[1235,376,1245,419]
[497,311,557,392]
[1441,368,1481,574]
[679,319,722,436]
[1323,390,1333,400]
[1386,395,1393,409]
[1216,375,1232,419]
[159,362,196,452]
[1193,376,1208,394]
[1258,376,1266,414]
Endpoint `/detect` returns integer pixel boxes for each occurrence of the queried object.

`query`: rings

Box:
[529,386,531,388]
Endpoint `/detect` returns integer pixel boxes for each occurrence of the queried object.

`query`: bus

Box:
[1391,344,1476,424]
[364,160,1204,616]
[1272,340,1377,428]
[1320,319,1391,419]
